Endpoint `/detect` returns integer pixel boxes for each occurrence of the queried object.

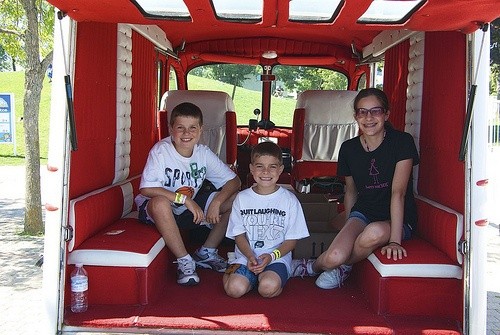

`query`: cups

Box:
[254,248,263,265]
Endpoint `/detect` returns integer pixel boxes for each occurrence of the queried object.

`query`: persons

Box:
[222,142,310,298]
[135,102,242,284]
[276,86,284,98]
[288,88,418,288]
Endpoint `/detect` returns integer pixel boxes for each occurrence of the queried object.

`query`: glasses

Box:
[356,107,387,117]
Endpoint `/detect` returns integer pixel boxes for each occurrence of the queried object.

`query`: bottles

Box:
[70,263,89,312]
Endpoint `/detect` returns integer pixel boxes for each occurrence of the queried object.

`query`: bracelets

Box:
[175,193,187,204]
[273,249,281,259]
[270,253,275,261]
[389,242,400,245]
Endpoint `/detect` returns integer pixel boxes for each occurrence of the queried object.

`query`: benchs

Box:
[67,168,172,309]
[361,189,463,318]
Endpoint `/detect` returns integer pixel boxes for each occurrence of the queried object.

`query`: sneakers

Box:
[315,264,353,290]
[193,248,230,272]
[291,258,321,278]
[172,259,200,286]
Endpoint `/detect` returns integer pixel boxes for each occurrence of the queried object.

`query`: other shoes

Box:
[227,252,235,265]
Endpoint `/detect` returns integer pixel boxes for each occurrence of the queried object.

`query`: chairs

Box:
[292,89,368,182]
[158,89,239,170]
